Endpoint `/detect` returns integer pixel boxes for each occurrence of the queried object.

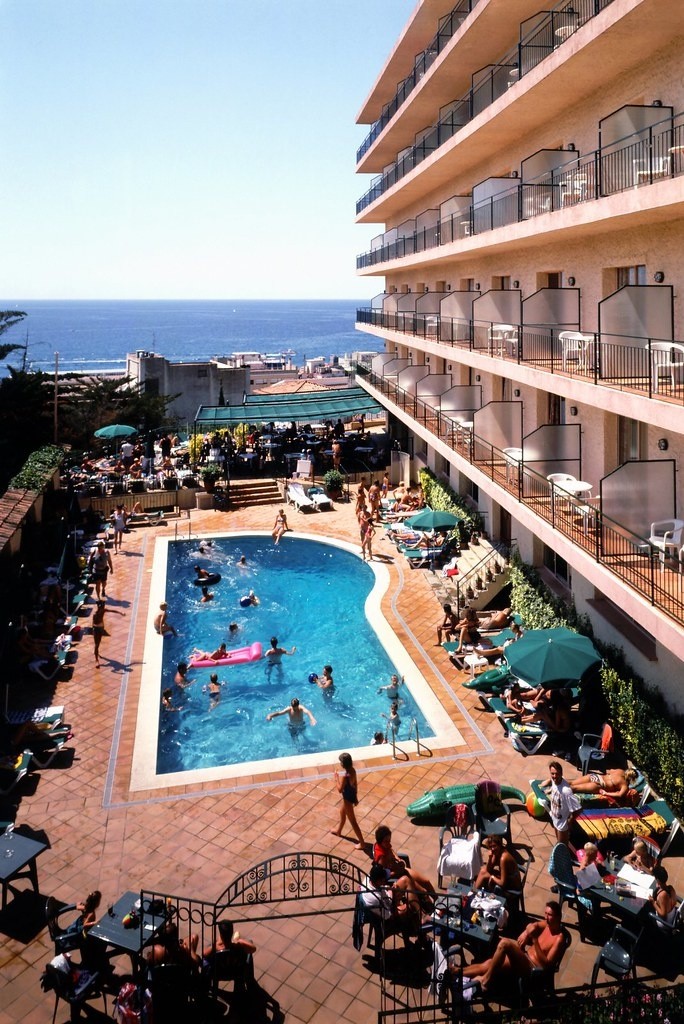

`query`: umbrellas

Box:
[404,511,465,560]
[94,424,138,459]
[503,627,603,690]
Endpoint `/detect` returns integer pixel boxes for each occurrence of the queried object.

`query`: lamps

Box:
[567,276,576,286]
[652,99,663,107]
[654,271,664,283]
[425,287,428,292]
[568,7,575,13]
[570,406,577,416]
[567,142,575,151]
[514,389,521,397]
[447,284,450,290]
[476,374,480,381]
[514,280,520,288]
[426,357,429,362]
[512,170,518,178]
[448,365,451,370]
[658,438,669,451]
[475,283,480,290]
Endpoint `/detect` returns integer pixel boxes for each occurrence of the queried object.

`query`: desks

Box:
[462,653,489,678]
[0,832,48,922]
[431,882,507,964]
[554,480,593,523]
[553,25,580,40]
[86,890,176,977]
[588,859,659,964]
[668,145,684,154]
[206,423,374,480]
[459,421,473,454]
[460,221,474,237]
[569,335,598,373]
[493,327,518,360]
[508,67,518,77]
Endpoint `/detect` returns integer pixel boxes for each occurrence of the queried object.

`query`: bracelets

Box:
[335,780,339,783]
[603,791,607,795]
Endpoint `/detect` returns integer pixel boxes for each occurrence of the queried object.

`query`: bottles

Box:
[481,887,487,898]
[607,850,615,862]
[167,897,172,910]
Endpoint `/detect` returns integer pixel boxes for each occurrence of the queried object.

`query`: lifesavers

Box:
[194,572,221,586]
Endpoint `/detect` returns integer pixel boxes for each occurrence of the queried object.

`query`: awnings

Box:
[193,395,388,473]
[243,387,368,404]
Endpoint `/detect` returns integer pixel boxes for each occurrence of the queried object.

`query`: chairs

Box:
[487,324,513,358]
[488,684,582,755]
[0,512,165,682]
[501,448,522,483]
[59,438,198,496]
[547,473,577,516]
[648,518,684,573]
[44,896,254,1024]
[559,179,587,209]
[645,342,684,397]
[441,609,525,668]
[445,417,465,449]
[0,706,71,792]
[285,484,333,513]
[352,767,684,1018]
[633,156,671,189]
[577,723,613,776]
[379,498,453,569]
[575,495,601,537]
[558,331,585,373]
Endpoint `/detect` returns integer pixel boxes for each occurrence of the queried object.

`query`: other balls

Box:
[240,596,251,607]
[308,673,318,684]
[525,791,546,818]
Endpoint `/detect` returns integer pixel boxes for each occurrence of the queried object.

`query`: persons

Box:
[575,841,677,933]
[538,767,638,798]
[259,637,296,682]
[79,412,368,480]
[194,565,214,603]
[0,721,71,751]
[248,590,260,607]
[109,503,134,546]
[192,541,212,559]
[370,675,405,745]
[549,762,582,843]
[160,643,231,713]
[266,698,317,744]
[271,508,291,545]
[229,622,238,633]
[313,665,335,707]
[76,890,257,968]
[9,542,125,668]
[335,753,366,850]
[434,604,570,733]
[355,472,448,562]
[236,556,249,575]
[154,602,186,637]
[360,826,567,989]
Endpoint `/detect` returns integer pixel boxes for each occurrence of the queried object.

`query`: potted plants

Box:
[188,433,205,472]
[200,463,224,493]
[458,520,487,550]
[452,564,501,608]
[322,467,346,500]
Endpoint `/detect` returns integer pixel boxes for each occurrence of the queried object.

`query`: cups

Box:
[450,873,457,887]
[481,917,491,933]
[610,857,615,871]
[107,902,114,917]
